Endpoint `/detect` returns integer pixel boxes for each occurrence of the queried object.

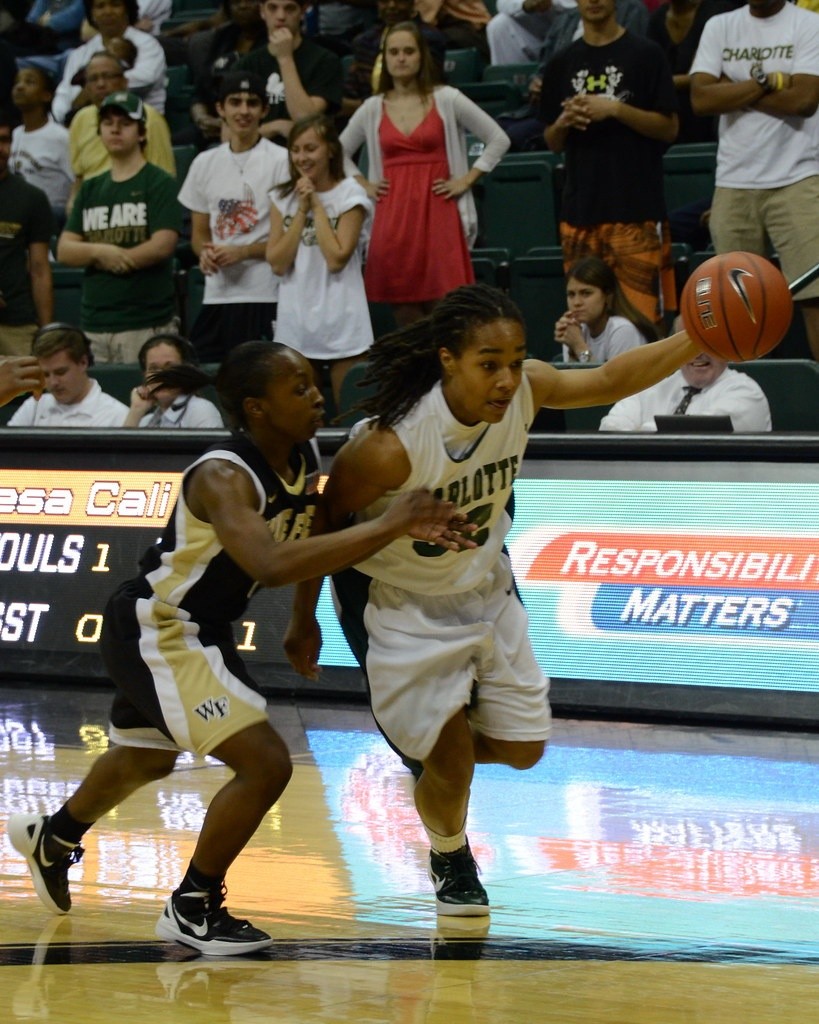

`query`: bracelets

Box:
[777,72,783,90]
[299,208,307,213]
[772,73,776,89]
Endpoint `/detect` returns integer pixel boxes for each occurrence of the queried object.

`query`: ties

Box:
[674,386,702,415]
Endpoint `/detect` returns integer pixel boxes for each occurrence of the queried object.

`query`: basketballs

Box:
[681,251,793,359]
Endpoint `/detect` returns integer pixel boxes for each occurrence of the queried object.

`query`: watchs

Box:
[757,74,771,93]
[577,350,592,362]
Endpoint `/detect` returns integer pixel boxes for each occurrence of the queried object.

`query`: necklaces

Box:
[230,152,250,174]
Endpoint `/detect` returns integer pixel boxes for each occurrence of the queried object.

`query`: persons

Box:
[0,113,55,356]
[1,0,708,230]
[554,256,659,364]
[56,91,185,361]
[7,321,130,426]
[268,115,374,417]
[177,72,290,361]
[283,283,703,918]
[689,0,819,358]
[539,0,680,333]
[7,340,477,958]
[597,314,772,432]
[337,22,511,328]
[0,355,46,407]
[122,334,224,428]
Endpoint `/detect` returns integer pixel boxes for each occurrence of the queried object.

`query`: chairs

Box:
[49,10,819,433]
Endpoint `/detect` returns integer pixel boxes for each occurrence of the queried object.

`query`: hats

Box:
[12,55,59,82]
[219,72,267,112]
[103,93,146,124]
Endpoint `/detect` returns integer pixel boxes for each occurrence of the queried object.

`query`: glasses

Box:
[86,72,122,84]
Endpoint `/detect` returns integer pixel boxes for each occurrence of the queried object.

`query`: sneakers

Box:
[7,812,84,915]
[29,916,81,1002]
[430,933,485,980]
[428,834,490,916]
[157,962,279,1019]
[157,880,272,956]
